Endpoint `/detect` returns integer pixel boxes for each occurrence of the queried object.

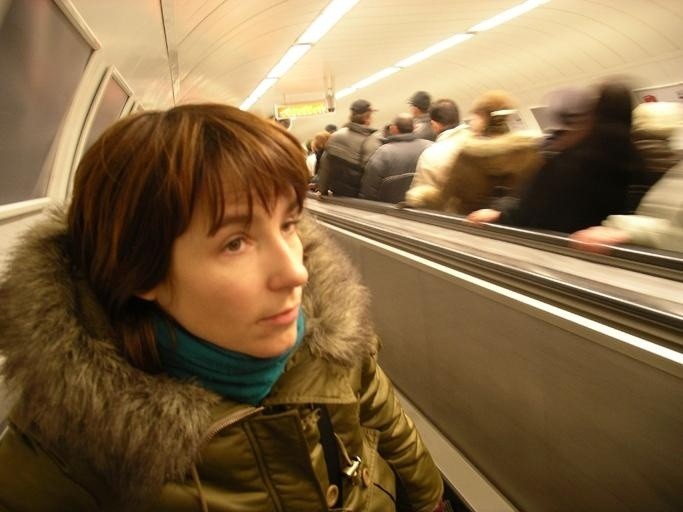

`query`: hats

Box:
[406,91,432,114]
[349,99,380,114]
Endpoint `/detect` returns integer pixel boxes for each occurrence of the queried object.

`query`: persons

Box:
[0,102,451,511]
[303,82,682,257]
